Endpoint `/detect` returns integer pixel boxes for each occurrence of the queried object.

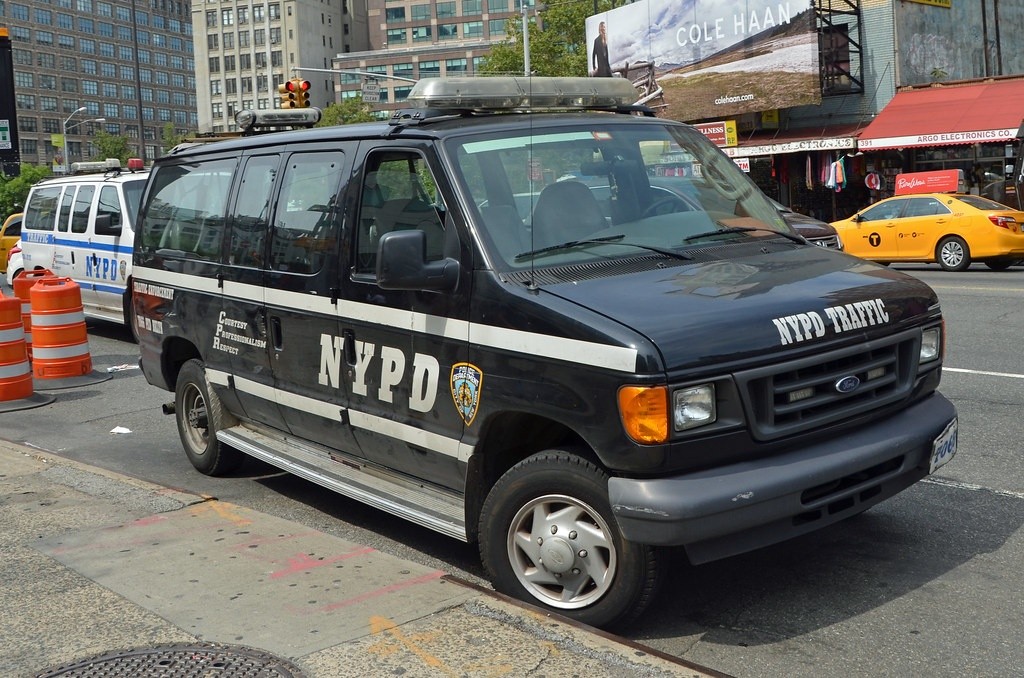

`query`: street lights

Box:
[62,106,106,171]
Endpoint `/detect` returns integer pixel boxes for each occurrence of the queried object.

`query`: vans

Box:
[0,212,49,272]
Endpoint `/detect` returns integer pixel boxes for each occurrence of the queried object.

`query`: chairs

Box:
[533,181,610,249]
[171,196,530,273]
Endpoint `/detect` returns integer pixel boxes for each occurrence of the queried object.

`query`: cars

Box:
[7,239,26,285]
[829,192,1024,272]
[474,193,540,223]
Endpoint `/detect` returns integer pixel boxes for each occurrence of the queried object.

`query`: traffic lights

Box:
[278,78,311,108]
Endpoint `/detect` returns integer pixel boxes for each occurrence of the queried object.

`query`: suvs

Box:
[523,175,845,252]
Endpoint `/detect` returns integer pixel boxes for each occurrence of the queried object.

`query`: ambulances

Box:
[131,76,959,635]
[21,158,150,344]
